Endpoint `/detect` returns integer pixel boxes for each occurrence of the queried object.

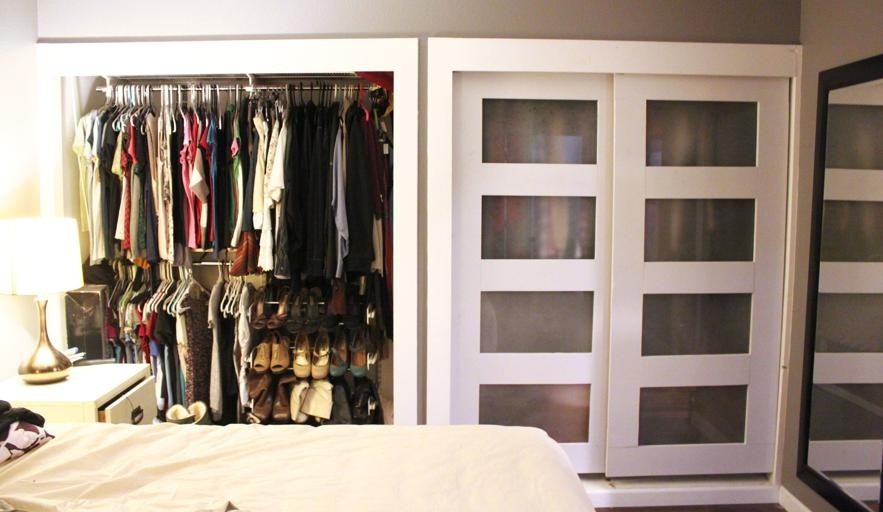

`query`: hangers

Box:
[91,82,386,135]
[91,244,385,322]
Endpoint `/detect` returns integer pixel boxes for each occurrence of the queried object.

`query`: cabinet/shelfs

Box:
[454,71,773,482]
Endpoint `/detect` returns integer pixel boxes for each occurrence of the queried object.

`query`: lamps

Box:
[4,219,84,383]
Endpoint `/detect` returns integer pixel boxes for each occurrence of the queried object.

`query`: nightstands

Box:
[10,363,158,425]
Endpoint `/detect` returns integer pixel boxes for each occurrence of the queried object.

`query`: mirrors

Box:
[796,52,883,512]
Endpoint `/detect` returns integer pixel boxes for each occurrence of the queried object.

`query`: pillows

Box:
[0,420,54,466]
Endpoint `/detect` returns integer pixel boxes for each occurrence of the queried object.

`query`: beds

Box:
[0,422,596,511]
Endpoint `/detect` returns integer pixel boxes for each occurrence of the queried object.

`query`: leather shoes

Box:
[245,278,382,424]
